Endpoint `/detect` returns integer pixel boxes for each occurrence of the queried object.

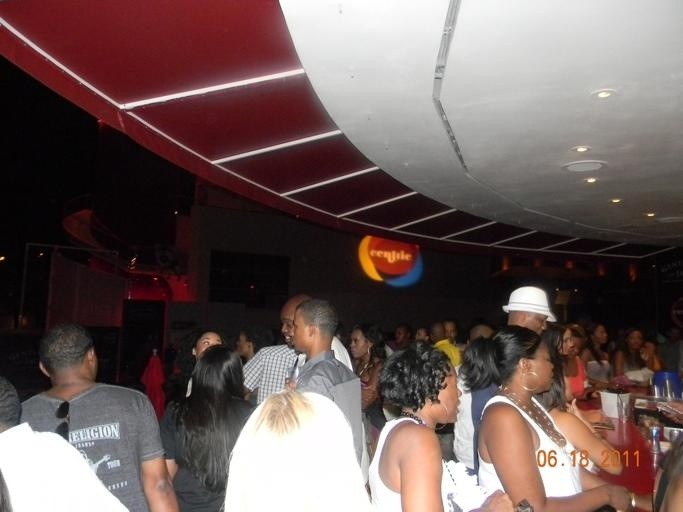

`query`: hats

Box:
[502,285,557,323]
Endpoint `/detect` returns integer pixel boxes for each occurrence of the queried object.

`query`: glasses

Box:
[54,400,71,443]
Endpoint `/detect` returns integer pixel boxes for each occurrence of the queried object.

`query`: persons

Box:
[471,287,557,474]
[615,432,683,512]
[415,328,429,341]
[243,344,297,405]
[460,326,631,512]
[0,375,131,512]
[429,323,462,367]
[469,326,492,339]
[368,339,518,512]
[350,325,384,437]
[581,325,611,383]
[224,389,373,512]
[237,331,262,406]
[159,345,254,512]
[567,325,584,359]
[444,320,467,350]
[535,343,623,474]
[385,324,412,356]
[656,325,683,378]
[613,329,660,381]
[174,327,229,400]
[20,324,180,512]
[541,324,587,403]
[291,298,363,466]
[282,294,354,391]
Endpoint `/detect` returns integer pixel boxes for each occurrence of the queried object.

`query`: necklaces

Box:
[357,359,374,377]
[500,384,567,446]
[400,411,457,486]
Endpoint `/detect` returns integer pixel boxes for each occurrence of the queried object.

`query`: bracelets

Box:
[630,492,638,512]
